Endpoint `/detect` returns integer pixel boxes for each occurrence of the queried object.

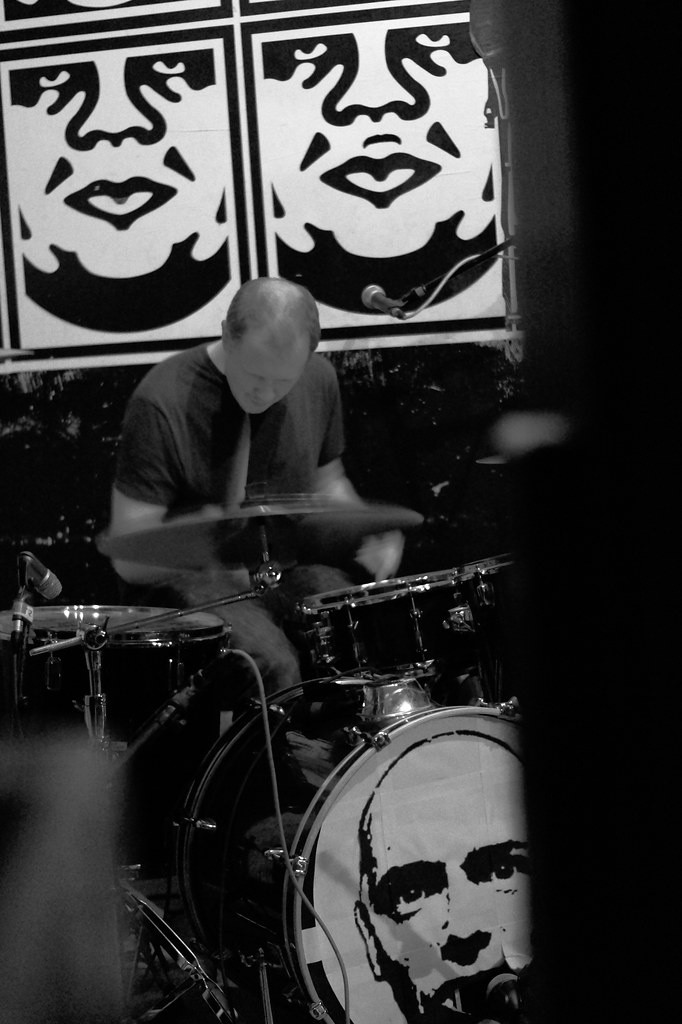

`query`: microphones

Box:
[487,973,521,1024]
[362,285,407,320]
[17,551,62,599]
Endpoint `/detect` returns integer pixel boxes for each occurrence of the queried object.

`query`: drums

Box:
[294,567,477,675]
[451,552,514,615]
[0,605,233,716]
[176,676,534,1024]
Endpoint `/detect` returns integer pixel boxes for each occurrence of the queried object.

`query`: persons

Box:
[110,278,406,726]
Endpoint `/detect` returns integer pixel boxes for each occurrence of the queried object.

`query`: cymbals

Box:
[95,500,424,573]
